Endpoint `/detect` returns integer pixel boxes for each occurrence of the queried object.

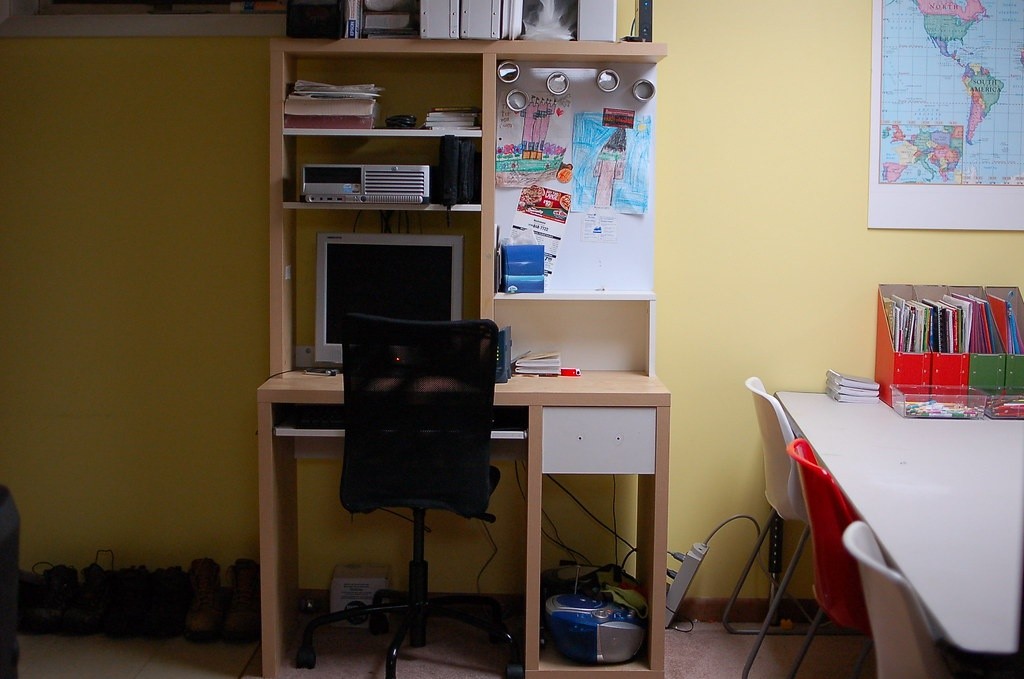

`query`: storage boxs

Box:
[285,0,617,39]
[890,384,1023,420]
[873,283,1024,410]
[330,565,392,629]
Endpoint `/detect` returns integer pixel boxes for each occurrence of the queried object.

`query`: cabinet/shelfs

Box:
[257,40,672,679]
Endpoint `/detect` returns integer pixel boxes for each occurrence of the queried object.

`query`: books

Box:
[825,369,881,405]
[418,106,482,130]
[883,293,1024,356]
[511,350,562,374]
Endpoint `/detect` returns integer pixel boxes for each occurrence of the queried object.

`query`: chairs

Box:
[720,372,947,679]
[296,311,524,679]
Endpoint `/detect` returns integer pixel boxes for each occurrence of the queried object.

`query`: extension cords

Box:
[665,542,710,627]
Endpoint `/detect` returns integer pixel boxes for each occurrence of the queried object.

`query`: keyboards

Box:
[295,414,346,429]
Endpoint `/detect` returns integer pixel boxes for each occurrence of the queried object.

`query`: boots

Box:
[219,558,262,645]
[181,556,226,642]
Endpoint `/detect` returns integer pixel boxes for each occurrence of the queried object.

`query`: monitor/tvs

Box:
[314,233,463,363]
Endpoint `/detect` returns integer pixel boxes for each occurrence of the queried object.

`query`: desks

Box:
[773,391,1023,656]
